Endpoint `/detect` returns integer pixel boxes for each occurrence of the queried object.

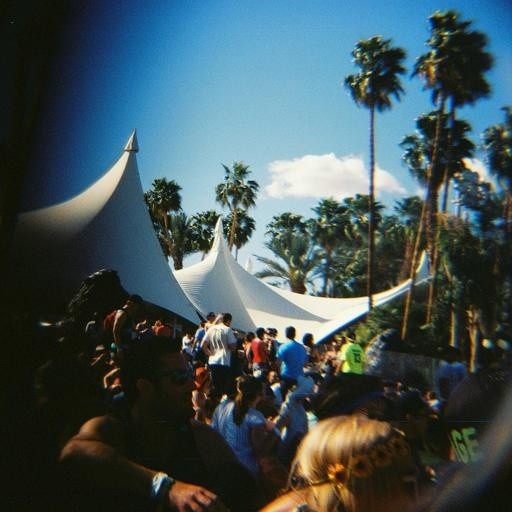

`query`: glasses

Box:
[177,363,195,387]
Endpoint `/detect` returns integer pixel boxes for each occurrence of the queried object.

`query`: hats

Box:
[345,333,356,342]
[130,294,144,304]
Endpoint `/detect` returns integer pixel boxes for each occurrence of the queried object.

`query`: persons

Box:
[1,267,512,510]
